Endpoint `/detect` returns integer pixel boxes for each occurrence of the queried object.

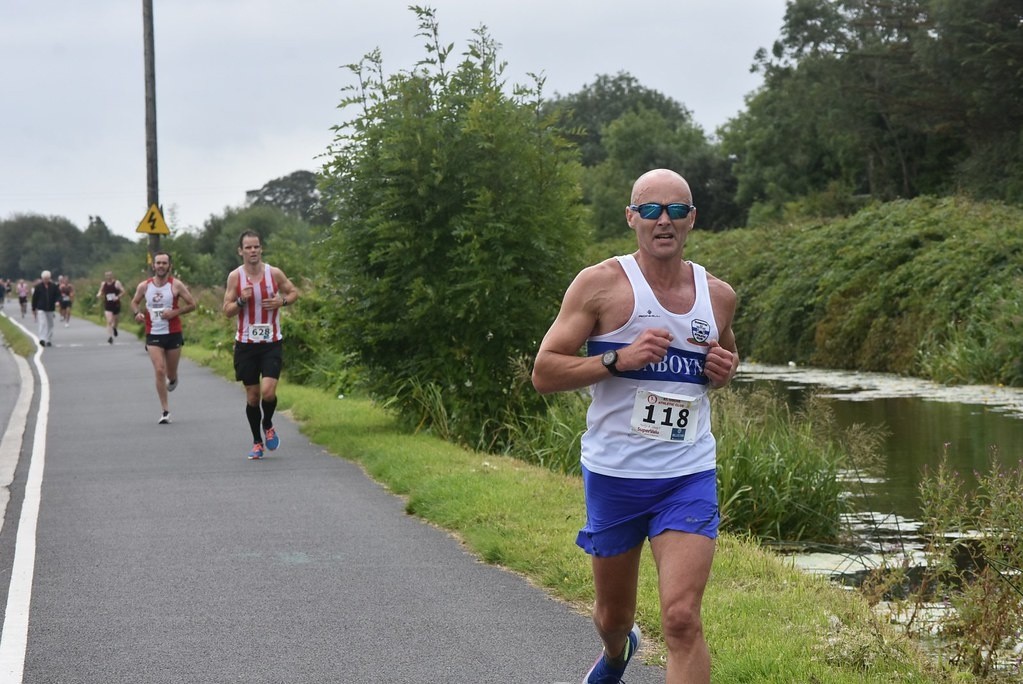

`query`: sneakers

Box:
[263,424,280,451]
[583,623,641,684]
[248,443,264,459]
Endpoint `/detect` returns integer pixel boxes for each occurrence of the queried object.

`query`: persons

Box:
[131,251,195,424]
[16,271,75,347]
[96,271,125,343]
[531,168,739,684]
[0,278,12,311]
[223,231,298,459]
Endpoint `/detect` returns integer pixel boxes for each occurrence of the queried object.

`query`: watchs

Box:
[602,349,622,377]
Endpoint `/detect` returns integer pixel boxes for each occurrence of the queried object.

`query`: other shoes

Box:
[65,324,69,327]
[113,329,117,337]
[47,342,50,347]
[166,376,178,392]
[40,340,45,347]
[158,410,171,424]
[107,337,112,344]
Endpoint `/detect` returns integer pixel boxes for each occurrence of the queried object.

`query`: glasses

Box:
[630,203,694,220]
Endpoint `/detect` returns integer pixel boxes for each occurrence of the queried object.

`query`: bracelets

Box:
[134,312,139,316]
[282,297,288,306]
[237,297,246,307]
